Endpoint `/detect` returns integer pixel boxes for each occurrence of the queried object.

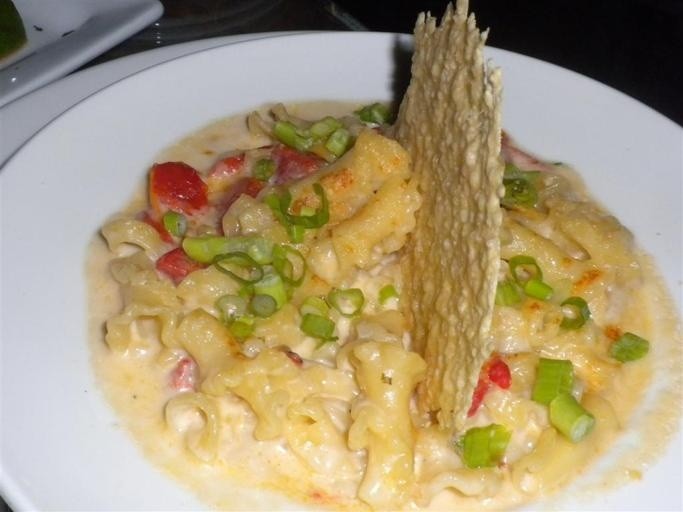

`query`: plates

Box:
[0,31,682,512]
[0,0,164,107]
